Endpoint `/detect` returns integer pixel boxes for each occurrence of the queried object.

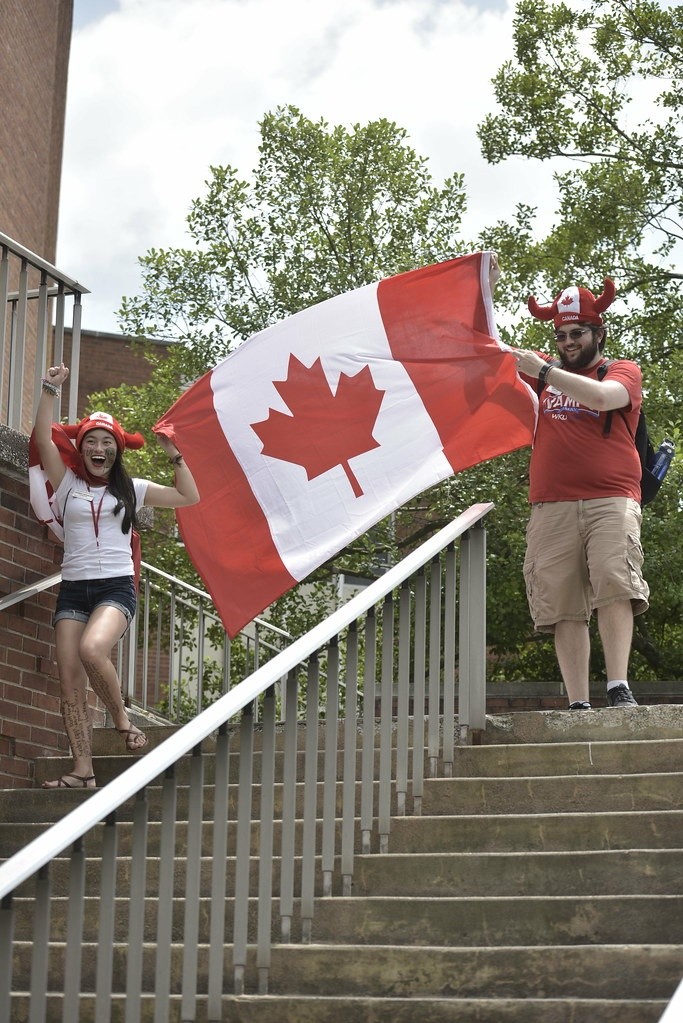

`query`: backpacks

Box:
[548,358,663,508]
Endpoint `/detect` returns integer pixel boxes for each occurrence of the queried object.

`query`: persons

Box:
[35,362,200,788]
[474,252,650,710]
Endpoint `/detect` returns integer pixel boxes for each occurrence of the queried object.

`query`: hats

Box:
[57,410,144,453]
[528,277,616,331]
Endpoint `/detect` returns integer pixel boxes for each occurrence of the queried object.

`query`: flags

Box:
[150,253,538,641]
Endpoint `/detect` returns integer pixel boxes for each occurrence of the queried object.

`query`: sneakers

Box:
[567,702,593,711]
[605,684,637,706]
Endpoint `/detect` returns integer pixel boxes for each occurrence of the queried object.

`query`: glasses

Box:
[553,327,594,343]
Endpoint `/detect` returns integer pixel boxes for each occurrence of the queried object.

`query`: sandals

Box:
[115,719,149,753]
[42,773,96,789]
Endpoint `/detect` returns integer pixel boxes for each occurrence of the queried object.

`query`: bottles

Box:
[647,438,676,482]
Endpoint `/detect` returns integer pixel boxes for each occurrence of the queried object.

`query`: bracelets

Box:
[539,363,554,384]
[40,378,61,397]
[168,454,183,467]
